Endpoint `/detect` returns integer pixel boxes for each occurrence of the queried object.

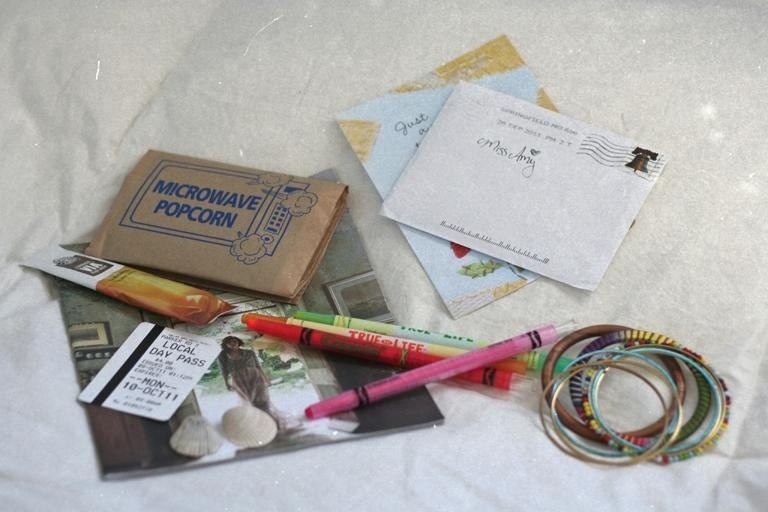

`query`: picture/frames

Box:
[322,269,395,324]
[67,320,113,350]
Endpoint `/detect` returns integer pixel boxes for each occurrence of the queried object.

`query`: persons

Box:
[216,336,281,434]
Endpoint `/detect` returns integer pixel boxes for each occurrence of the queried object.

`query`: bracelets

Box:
[538,323,730,466]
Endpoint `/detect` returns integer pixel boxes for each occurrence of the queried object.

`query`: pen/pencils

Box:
[304,319,578,420]
[247,317,540,397]
[241,311,526,375]
[294,310,577,376]
